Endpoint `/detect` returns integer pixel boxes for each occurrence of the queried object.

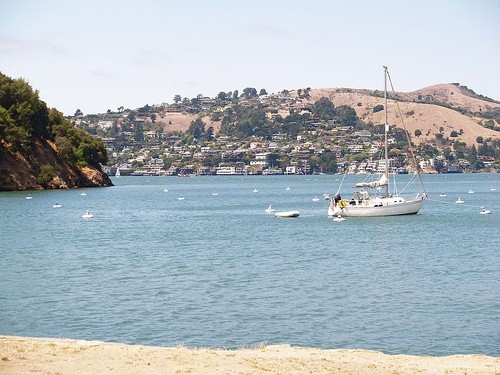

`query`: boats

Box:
[164,188,168,192]
[456,197,464,204]
[82,210,93,218]
[468,188,475,193]
[178,195,185,200]
[26,194,32,199]
[323,191,331,200]
[265,205,275,213]
[490,188,496,191]
[53,201,62,208]
[312,194,319,202]
[285,185,291,191]
[479,205,491,215]
[275,210,299,217]
[253,187,258,192]
[81,191,87,196]
[439,191,446,197]
[328,65,429,222]
[212,191,220,196]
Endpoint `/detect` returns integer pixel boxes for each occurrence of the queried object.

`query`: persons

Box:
[350,198,356,205]
[335,193,344,206]
